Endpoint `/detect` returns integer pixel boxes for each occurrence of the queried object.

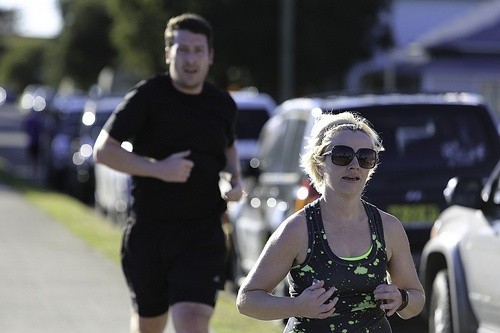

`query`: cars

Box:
[0,95,125,209]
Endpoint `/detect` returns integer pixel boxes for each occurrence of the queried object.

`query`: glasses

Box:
[322,145,378,169]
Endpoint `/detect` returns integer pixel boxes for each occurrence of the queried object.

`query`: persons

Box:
[236,116,426,333]
[93,14,242,333]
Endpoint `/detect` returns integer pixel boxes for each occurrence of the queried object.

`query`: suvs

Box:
[223,91,500,325]
[89,87,278,225]
[416,162,500,333]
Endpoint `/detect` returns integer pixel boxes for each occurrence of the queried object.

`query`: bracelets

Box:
[396,288,409,312]
